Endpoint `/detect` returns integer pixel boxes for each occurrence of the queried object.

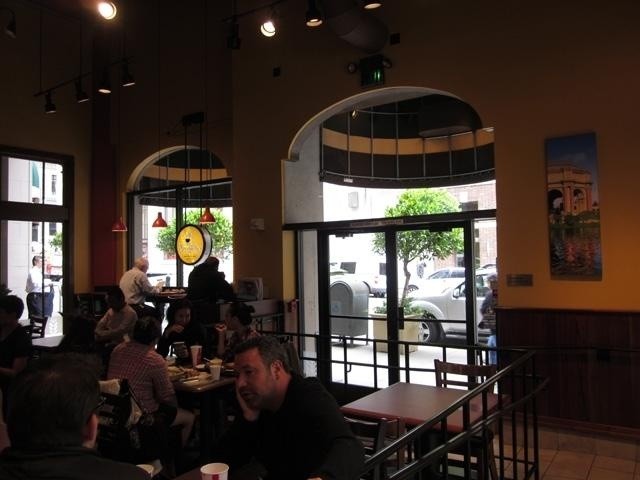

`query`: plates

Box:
[168,370,184,381]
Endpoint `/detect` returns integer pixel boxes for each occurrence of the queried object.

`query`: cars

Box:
[331,257,499,345]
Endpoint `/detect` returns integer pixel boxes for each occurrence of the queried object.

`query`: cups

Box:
[136,464,155,480]
[173,342,184,355]
[190,345,202,369]
[210,365,222,381]
[200,462,229,480]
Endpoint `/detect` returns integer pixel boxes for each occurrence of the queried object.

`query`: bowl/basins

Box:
[209,358,223,365]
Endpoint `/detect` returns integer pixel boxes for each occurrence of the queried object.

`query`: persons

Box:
[480,273,498,393]
[208,335,364,480]
[1,254,265,479]
